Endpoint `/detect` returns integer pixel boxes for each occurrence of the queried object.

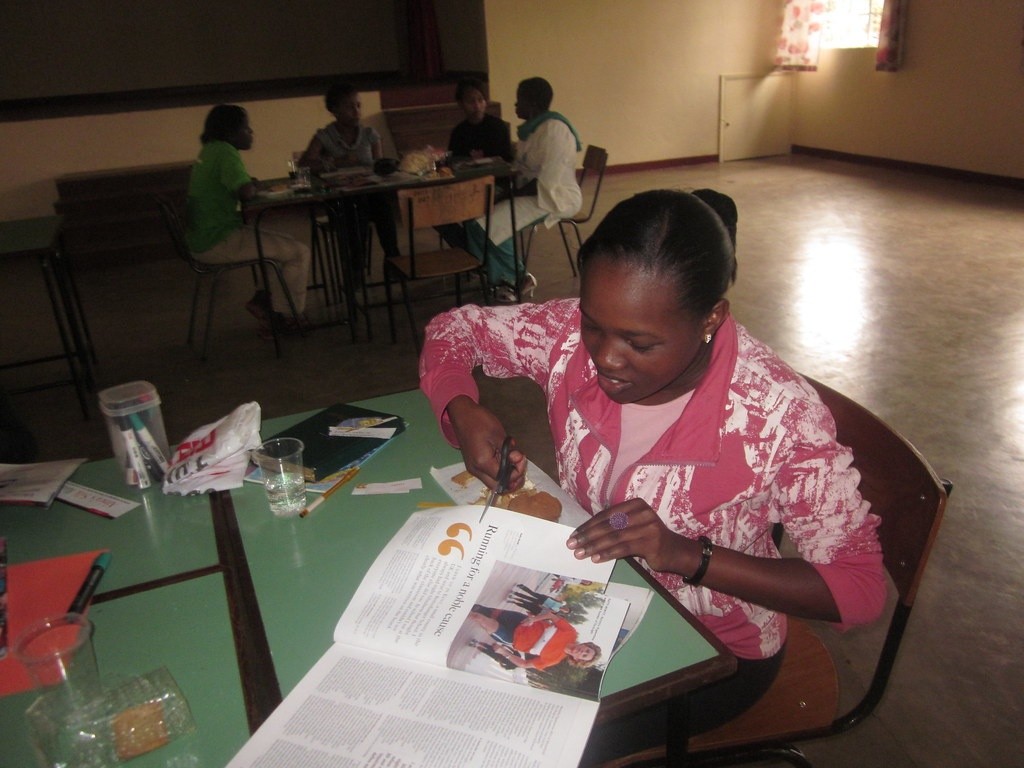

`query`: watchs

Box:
[506,654,512,659]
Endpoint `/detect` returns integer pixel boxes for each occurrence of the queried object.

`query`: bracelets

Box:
[683,537,713,583]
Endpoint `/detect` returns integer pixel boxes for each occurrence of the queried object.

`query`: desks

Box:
[0,214,98,422]
[240,152,526,358]
[0,383,739,768]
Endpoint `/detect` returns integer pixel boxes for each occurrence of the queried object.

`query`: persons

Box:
[295,82,403,292]
[183,105,310,340]
[430,78,512,253]
[461,77,584,303]
[421,189,885,768]
[467,574,602,669]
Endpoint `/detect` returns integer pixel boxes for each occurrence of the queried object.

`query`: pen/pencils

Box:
[298,467,362,520]
[285,160,296,171]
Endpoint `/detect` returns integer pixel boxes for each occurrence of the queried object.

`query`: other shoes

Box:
[247,291,313,341]
[490,271,538,301]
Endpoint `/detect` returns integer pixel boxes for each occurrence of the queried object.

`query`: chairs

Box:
[580,365,953,768]
[146,121,609,359]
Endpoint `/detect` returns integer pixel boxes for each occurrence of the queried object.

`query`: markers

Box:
[108,394,168,489]
[68,554,110,618]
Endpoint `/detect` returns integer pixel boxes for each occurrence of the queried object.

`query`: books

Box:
[242,403,409,494]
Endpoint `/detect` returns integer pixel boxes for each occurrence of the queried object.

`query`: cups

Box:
[285,158,312,192]
[98,379,172,490]
[10,613,197,767]
[252,437,306,518]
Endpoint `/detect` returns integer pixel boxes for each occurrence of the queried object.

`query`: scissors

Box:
[479,435,518,523]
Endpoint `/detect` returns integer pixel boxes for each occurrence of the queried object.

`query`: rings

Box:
[610,511,629,530]
